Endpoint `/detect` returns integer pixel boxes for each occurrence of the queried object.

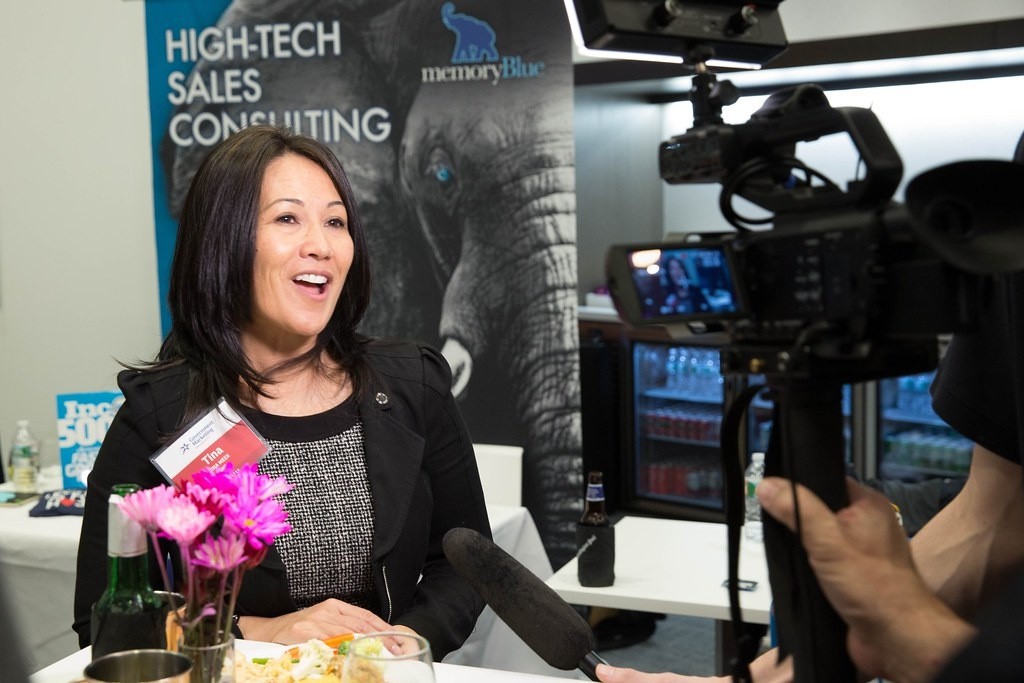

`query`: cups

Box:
[341,632,436,683]
[153,591,186,652]
[83,650,193,683]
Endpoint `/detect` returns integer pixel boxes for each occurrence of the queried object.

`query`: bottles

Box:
[13,420,36,491]
[897,371,935,417]
[743,452,764,541]
[579,470,611,528]
[664,348,723,397]
[91,484,163,614]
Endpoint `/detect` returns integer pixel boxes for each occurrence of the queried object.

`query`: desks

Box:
[544,514,774,683]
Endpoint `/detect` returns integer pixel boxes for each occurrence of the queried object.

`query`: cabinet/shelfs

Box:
[617,323,976,538]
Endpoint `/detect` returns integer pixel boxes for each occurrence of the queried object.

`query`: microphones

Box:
[442,527,612,682]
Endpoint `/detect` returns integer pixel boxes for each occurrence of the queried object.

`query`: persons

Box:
[654,257,713,313]
[595,131,1024,683]
[71,130,492,665]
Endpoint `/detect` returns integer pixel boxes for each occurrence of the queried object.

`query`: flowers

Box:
[115,458,290,648]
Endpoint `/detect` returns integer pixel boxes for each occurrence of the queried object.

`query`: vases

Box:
[176,628,235,681]
